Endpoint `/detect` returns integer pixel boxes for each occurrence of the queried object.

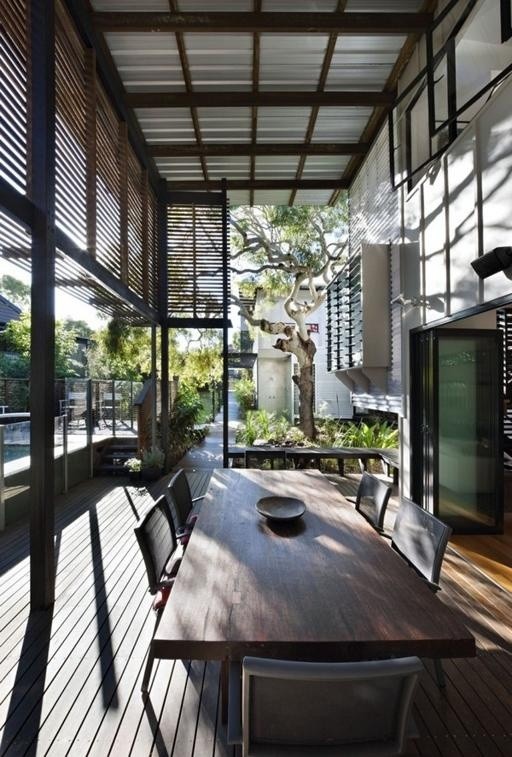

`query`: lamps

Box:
[390,292,430,308]
[469,245,512,280]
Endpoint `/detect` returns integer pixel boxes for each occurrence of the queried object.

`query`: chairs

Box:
[132,495,191,696]
[60,391,89,430]
[223,653,424,757]
[98,392,123,427]
[243,450,289,471]
[167,469,207,534]
[375,495,454,696]
[343,469,392,533]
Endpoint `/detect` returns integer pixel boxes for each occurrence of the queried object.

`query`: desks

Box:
[151,467,478,729]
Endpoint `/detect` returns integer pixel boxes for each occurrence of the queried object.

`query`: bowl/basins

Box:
[255,496,308,520]
